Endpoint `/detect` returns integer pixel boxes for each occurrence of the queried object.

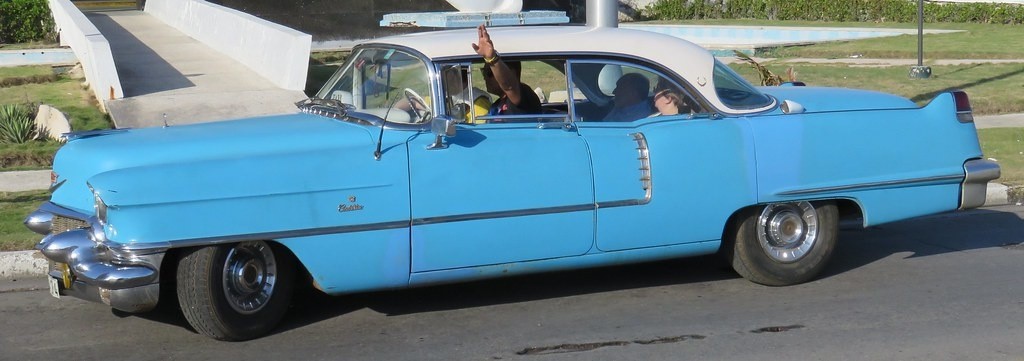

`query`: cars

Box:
[25,22,1003,342]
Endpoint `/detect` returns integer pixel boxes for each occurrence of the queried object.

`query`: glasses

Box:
[480,67,492,76]
[653,85,660,92]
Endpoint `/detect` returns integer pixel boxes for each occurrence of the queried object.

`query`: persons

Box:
[648,76,687,119]
[469,22,544,117]
[390,64,492,126]
[599,73,659,123]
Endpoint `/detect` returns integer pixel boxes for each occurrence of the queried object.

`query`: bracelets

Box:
[484,50,501,65]
